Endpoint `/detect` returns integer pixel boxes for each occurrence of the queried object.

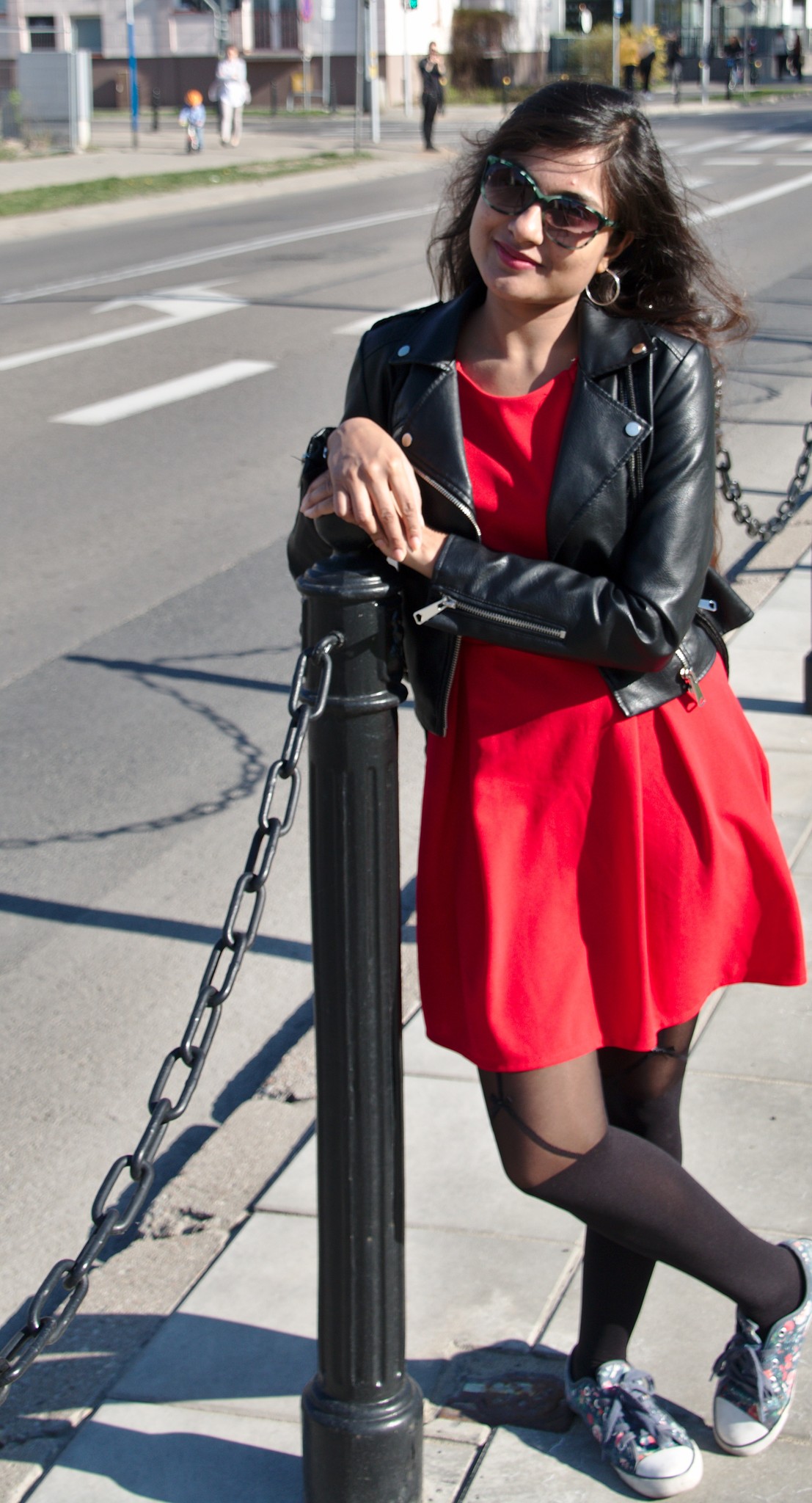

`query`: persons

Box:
[790,28,805,79]
[179,90,207,152]
[418,41,446,152]
[214,45,251,148]
[284,78,811,1498]
[666,36,689,103]
[771,27,788,81]
[618,28,655,100]
[724,37,743,100]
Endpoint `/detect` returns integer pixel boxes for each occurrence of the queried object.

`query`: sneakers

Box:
[708,1238,812,1454]
[563,1344,703,1498]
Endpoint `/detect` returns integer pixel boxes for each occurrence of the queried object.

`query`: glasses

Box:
[481,154,617,252]
